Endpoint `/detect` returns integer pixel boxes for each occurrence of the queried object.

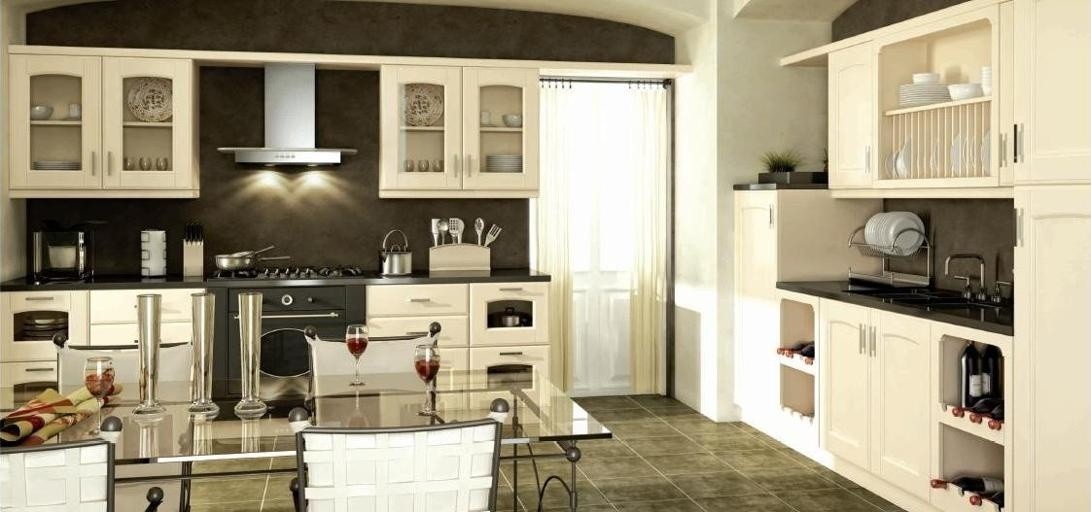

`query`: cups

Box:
[481,110,490,124]
[403,158,443,171]
[122,156,168,171]
[69,104,81,118]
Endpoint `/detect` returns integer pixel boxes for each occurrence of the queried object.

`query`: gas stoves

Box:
[207,267,363,308]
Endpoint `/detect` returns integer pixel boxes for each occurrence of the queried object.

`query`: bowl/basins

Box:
[945,84,981,102]
[981,64,993,95]
[30,107,53,121]
[913,73,942,85]
[504,114,524,127]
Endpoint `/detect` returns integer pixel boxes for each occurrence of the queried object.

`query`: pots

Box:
[491,306,532,327]
[215,244,292,269]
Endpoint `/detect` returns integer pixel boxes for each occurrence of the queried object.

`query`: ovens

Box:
[212,309,365,400]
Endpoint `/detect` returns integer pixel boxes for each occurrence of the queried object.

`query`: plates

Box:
[898,82,950,107]
[864,211,925,256]
[399,84,444,127]
[486,153,521,172]
[22,315,68,338]
[128,77,173,121]
[31,159,81,171]
[886,129,991,181]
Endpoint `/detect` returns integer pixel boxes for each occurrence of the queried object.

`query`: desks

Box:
[0,373,613,512]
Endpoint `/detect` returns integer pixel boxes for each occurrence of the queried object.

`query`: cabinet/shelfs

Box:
[732,183,884,462]
[0,288,206,479]
[1014,0,1089,183]
[869,0,1000,200]
[929,319,1012,512]
[826,28,873,198]
[366,281,550,373]
[779,288,819,462]
[819,296,929,512]
[7,45,200,199]
[1012,185,1091,512]
[379,55,540,199]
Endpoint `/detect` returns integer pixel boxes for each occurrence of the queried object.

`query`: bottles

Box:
[777,338,814,359]
[931,472,1006,507]
[959,340,1005,422]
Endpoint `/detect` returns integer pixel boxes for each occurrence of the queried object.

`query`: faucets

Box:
[944,253,988,302]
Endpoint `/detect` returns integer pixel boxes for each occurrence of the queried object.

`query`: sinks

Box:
[892,299,1002,311]
[842,285,959,300]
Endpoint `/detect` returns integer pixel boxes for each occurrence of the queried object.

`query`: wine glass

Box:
[346,324,368,384]
[414,345,443,415]
[82,355,116,436]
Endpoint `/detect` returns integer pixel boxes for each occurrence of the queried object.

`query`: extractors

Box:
[217,62,360,168]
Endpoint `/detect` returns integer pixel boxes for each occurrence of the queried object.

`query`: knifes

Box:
[184,218,204,245]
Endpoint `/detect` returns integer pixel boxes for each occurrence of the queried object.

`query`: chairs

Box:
[302,322,441,375]
[0,416,163,512]
[52,325,195,372]
[288,397,509,512]
